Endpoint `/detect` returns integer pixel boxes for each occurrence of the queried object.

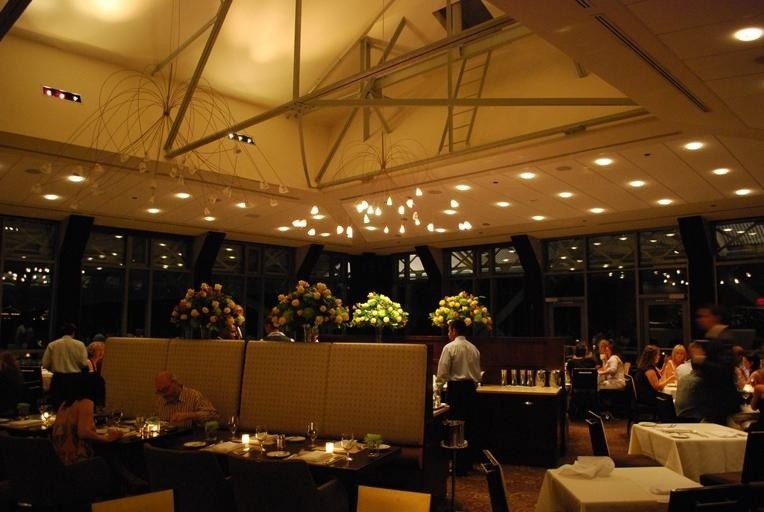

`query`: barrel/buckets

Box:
[442,442,468,475]
[443,419,467,446]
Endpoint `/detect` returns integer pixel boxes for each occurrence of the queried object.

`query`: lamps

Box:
[305,0,474,241]
[21,0,288,224]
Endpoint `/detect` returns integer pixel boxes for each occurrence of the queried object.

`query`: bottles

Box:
[276,432,287,449]
[305,421,317,447]
[226,416,236,440]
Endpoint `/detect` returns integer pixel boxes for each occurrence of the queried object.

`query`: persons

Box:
[15,319,32,346]
[153,371,221,427]
[435,319,482,419]
[0,350,37,414]
[264,316,286,337]
[567,305,764,432]
[51,374,124,466]
[41,323,89,413]
[87,341,104,375]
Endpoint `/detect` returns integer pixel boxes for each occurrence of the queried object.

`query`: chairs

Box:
[572,368,602,422]
[586,412,658,466]
[624,374,658,437]
[480,450,510,511]
[667,484,763,511]
[700,422,764,487]
[656,392,706,420]
[357,486,431,511]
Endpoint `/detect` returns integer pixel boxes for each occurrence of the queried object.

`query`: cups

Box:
[500,369,533,386]
[367,440,380,457]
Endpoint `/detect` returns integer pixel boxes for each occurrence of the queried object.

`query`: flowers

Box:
[169,281,245,340]
[345,289,411,333]
[427,289,496,333]
[270,278,352,333]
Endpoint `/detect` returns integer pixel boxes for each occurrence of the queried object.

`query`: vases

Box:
[303,324,319,343]
[200,325,217,340]
[370,328,384,343]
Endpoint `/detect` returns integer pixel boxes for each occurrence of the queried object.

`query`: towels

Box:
[650,481,704,494]
[555,456,616,478]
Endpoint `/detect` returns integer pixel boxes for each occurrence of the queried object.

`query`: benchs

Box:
[238,342,431,484]
[101,337,245,428]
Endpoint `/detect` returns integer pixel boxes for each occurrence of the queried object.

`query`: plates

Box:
[266,450,291,458]
[286,436,306,442]
[367,444,391,449]
[184,441,206,448]
[638,422,656,427]
[672,433,689,438]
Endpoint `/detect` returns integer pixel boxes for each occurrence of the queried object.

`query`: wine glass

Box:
[341,432,355,461]
[255,425,268,455]
[136,416,144,437]
